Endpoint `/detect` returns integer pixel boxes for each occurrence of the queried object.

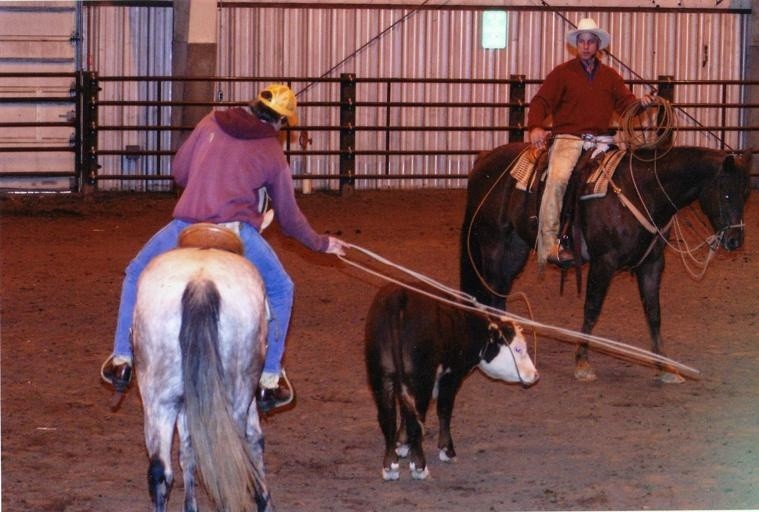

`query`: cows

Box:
[366,282,539,482]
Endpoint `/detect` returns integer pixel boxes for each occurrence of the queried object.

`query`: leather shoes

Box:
[103,357,132,391]
[548,250,575,266]
[256,383,291,412]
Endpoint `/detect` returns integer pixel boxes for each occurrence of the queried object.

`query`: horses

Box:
[132,187,274,512]
[459,142,755,385]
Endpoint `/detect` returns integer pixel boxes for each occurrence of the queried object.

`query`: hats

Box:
[258,84,299,126]
[567,18,610,50]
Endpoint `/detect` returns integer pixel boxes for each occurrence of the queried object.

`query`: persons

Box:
[527,19,659,264]
[97,82,353,418]
